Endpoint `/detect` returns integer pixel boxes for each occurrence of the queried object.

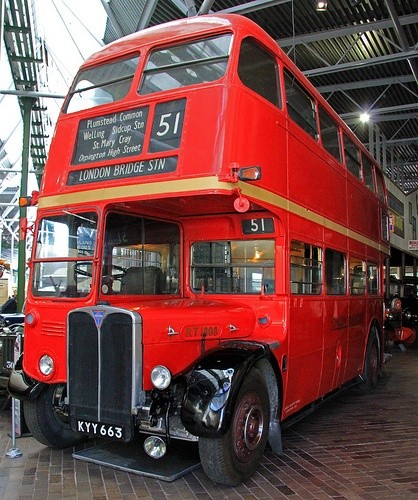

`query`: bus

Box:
[1,12,392,488]
